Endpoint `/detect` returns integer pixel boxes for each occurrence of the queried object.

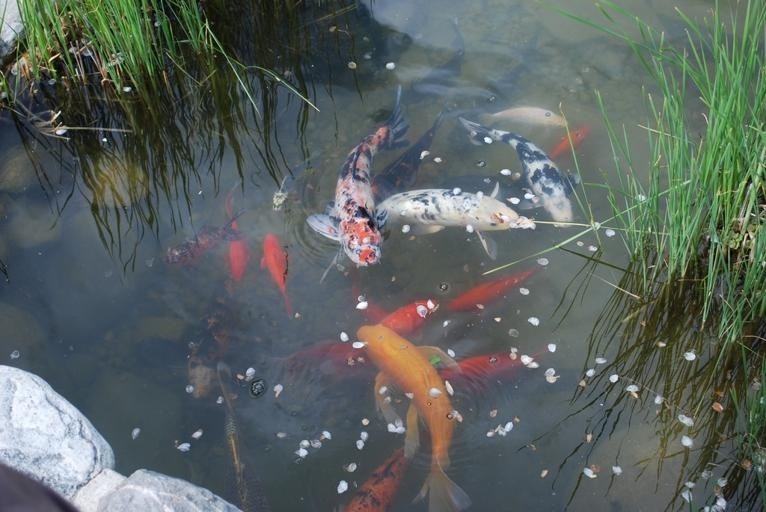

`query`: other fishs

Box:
[164,20,590,512]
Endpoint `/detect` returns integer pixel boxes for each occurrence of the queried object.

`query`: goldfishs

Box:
[164,20,590,512]
